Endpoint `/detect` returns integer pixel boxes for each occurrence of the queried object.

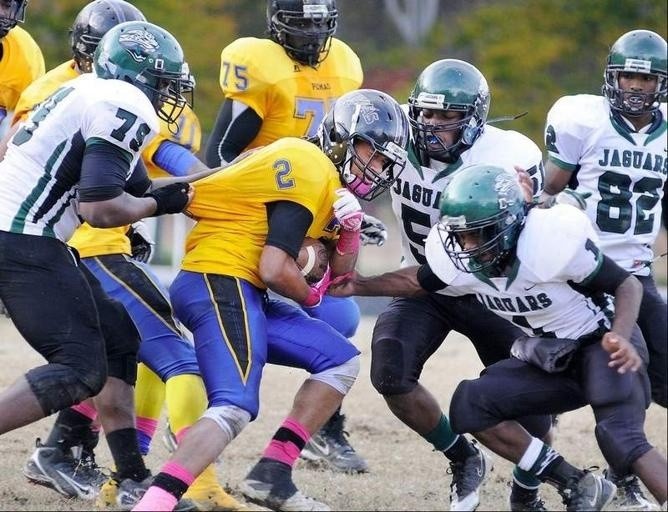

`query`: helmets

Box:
[90,19,184,78]
[602,28,668,78]
[266,0,335,21]
[67,0,148,70]
[408,59,491,108]
[437,162,526,222]
[318,88,409,153]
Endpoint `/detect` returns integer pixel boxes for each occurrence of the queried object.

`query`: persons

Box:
[0,20,198,511]
[66,95,243,512]
[542,29,668,510]
[204,1,388,475]
[323,164,668,512]
[131,88,409,511]
[369,58,554,512]
[0,0,47,141]
[0,0,149,503]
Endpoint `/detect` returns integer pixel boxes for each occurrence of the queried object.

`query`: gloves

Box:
[333,184,363,258]
[361,210,388,246]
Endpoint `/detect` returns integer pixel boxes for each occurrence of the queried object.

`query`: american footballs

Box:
[297,237,329,282]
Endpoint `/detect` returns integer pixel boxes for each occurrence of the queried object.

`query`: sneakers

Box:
[603,469,659,511]
[23,437,109,501]
[300,417,368,473]
[234,468,332,512]
[444,436,495,511]
[117,468,197,512]
[560,468,617,512]
[508,489,547,512]
[182,480,247,510]
[99,472,121,507]
[163,425,179,453]
